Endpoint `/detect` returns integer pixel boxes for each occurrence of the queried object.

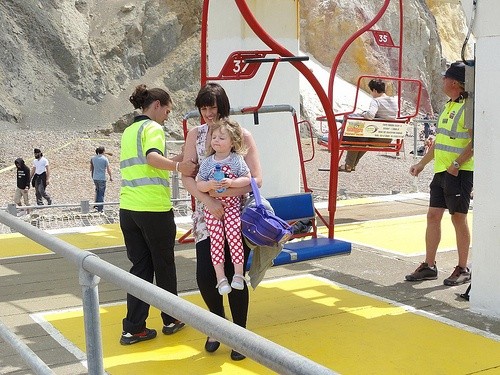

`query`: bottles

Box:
[213,165,226,194]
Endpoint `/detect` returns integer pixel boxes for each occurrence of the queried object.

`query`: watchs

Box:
[452,160,460,169]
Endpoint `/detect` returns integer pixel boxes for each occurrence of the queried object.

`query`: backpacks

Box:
[240,178,290,247]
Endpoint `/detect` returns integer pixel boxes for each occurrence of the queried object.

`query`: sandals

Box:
[338,164,351,172]
[351,166,356,171]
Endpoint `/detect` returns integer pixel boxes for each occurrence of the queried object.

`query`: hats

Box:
[34,149,40,153]
[441,62,465,84]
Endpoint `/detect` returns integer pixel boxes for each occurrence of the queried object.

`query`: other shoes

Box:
[231,349,245,360]
[95,206,99,210]
[27,208,31,214]
[230,274,247,290]
[205,337,220,352]
[216,276,232,295]
[48,200,51,205]
[99,210,104,213]
[21,208,26,214]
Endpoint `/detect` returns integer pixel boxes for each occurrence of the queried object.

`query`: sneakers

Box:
[120,328,157,345]
[405,261,438,281]
[444,266,471,285]
[162,320,185,335]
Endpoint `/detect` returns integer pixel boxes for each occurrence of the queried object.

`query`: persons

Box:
[339,78,399,172]
[30,148,52,205]
[405,60,474,286]
[119,85,197,346]
[14,157,31,219]
[198,118,252,296]
[178,83,262,361]
[90,147,113,211]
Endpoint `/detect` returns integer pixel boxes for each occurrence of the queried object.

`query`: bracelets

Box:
[176,161,179,172]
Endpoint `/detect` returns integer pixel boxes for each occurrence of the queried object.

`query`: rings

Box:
[191,159,194,162]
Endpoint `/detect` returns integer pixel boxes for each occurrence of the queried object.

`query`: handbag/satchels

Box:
[241,190,277,250]
